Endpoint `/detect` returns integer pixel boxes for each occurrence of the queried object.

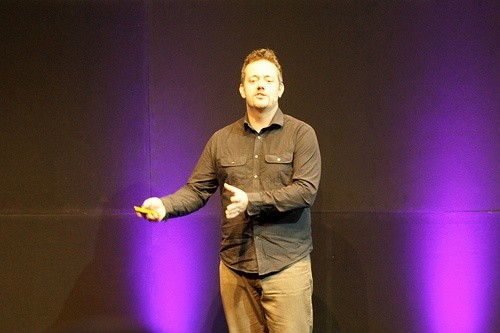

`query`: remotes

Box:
[134,206,161,216]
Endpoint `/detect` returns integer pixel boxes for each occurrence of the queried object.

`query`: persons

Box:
[135,49,324,333]
